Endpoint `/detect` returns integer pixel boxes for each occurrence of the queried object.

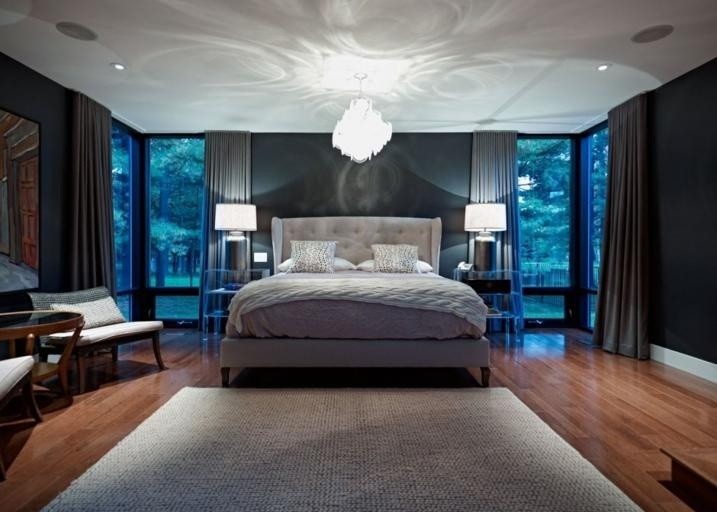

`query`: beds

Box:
[216,216,492,388]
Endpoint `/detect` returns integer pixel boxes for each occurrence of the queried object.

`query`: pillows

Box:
[356,258,433,274]
[277,256,356,271]
[286,239,338,273]
[369,244,422,275]
[49,297,124,330]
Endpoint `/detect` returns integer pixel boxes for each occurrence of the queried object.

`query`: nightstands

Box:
[454,269,519,333]
[200,269,271,340]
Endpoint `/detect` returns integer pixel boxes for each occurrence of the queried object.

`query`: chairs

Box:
[28,286,166,396]
[0,355,44,482]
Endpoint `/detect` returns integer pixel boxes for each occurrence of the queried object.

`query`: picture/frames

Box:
[0,107,42,297]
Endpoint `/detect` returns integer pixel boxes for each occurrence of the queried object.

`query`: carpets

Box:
[39,383,645,511]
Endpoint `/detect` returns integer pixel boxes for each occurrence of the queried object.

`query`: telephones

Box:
[457,261,474,271]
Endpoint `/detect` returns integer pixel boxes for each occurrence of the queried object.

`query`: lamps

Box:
[331,72,393,165]
[213,203,257,271]
[464,203,506,272]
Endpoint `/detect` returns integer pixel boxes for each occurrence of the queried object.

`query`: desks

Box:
[0,309,84,416]
